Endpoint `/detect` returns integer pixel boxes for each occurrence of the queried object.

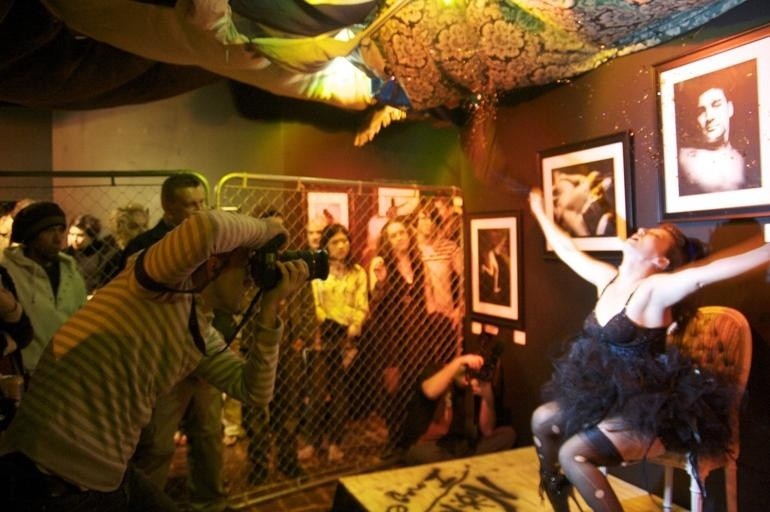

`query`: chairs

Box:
[647,302,753,512]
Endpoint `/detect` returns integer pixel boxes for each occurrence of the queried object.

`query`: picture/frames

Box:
[462,206,526,333]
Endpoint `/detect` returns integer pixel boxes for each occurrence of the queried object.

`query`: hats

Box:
[12,202,66,245]
[69,213,100,237]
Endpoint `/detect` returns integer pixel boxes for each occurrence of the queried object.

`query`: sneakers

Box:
[248,444,345,483]
[380,440,408,459]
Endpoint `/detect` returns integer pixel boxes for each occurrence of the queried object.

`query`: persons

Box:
[392,338,520,466]
[516,187,769,511]
[679,80,756,193]
[1,173,465,511]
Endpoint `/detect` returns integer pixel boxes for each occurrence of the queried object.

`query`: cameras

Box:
[470,356,498,384]
[249,204,332,294]
